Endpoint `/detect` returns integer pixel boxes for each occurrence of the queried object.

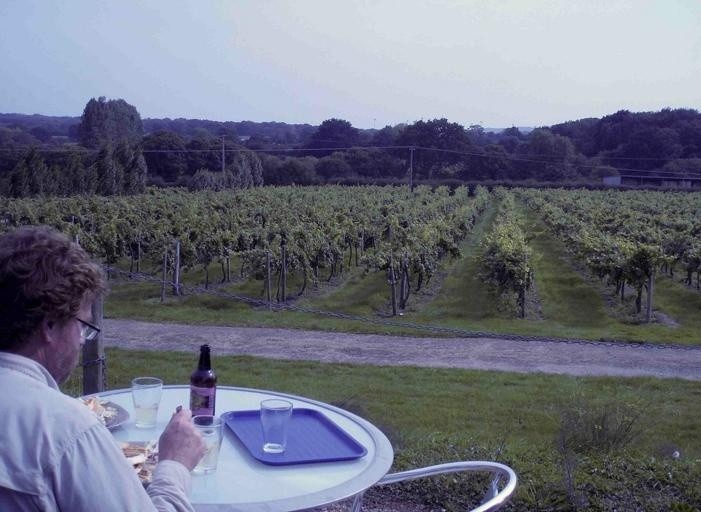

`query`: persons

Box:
[1,223,208,511]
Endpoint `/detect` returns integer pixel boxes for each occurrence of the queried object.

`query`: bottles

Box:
[188,344,217,425]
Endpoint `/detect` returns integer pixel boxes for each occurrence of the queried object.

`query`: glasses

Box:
[68,308,100,344]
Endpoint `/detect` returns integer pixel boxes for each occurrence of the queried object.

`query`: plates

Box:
[101,400,130,429]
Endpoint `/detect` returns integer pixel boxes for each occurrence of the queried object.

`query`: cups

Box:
[132,377,163,428]
[260,399,293,453]
[190,415,225,475]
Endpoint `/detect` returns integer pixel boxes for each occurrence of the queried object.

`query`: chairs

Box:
[350,461,518,512]
